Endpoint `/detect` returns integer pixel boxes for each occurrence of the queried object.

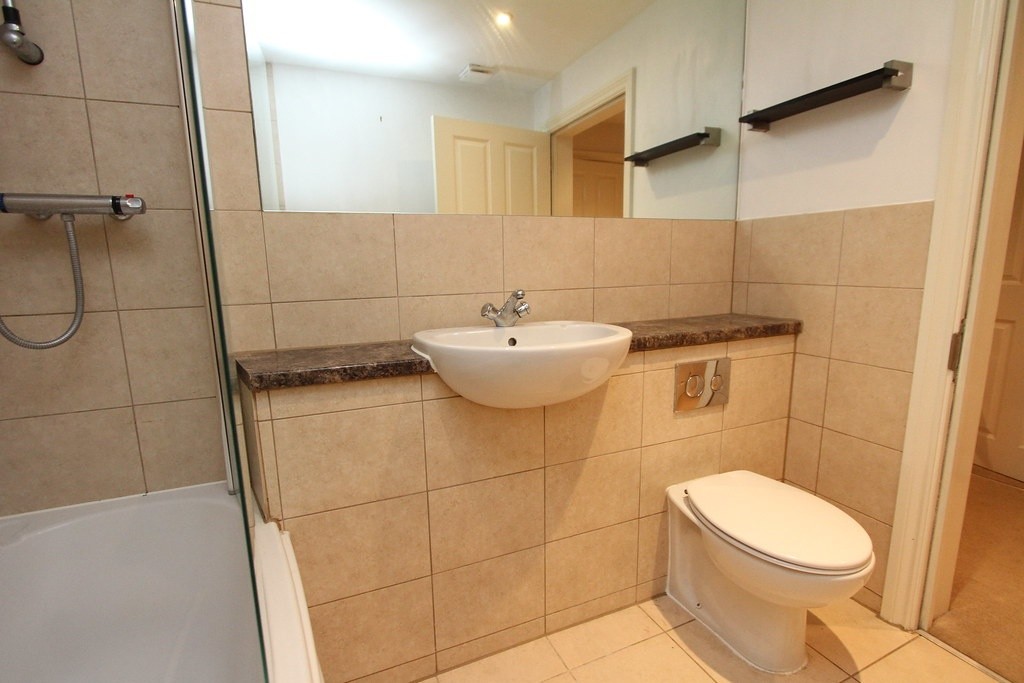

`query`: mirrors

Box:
[239,1,748,221]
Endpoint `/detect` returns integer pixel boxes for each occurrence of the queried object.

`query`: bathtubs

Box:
[0,480,329,682]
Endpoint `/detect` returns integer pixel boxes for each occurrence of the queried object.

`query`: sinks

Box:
[414,320,633,407]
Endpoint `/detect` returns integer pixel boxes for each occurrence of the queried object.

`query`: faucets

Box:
[480,289,530,327]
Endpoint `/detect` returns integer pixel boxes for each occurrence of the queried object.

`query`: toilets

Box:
[667,468,878,671]
